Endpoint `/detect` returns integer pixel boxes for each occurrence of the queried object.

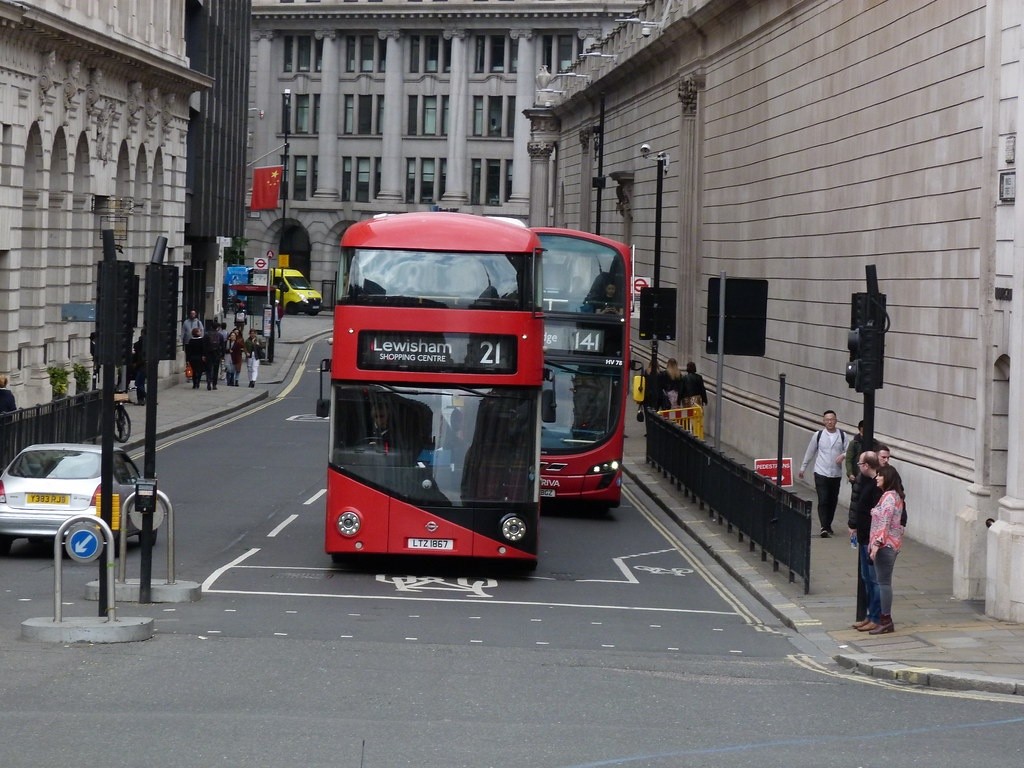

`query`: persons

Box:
[89,332,102,391]
[356,402,409,450]
[846,421,908,635]
[0,375,18,468]
[275,299,283,338]
[181,302,264,390]
[637,358,708,437]
[134,329,147,406]
[798,409,851,538]
[596,282,623,316]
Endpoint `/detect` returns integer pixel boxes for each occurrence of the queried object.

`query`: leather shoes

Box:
[852,619,869,628]
[857,621,877,631]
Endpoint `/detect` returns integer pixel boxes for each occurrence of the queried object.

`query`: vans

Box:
[248,268,323,316]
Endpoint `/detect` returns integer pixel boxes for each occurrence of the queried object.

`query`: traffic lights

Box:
[846,325,864,394]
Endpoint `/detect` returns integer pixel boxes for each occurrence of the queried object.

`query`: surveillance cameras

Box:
[642,27,651,38]
[545,101,551,108]
[640,144,650,154]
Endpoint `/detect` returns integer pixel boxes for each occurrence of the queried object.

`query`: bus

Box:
[315,212,543,576]
[528,226,646,516]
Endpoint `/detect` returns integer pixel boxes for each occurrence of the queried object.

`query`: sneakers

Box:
[821,528,828,538]
[827,526,834,535]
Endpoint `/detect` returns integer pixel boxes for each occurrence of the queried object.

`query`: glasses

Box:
[857,462,865,465]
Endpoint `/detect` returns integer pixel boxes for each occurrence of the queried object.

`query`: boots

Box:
[869,614,894,634]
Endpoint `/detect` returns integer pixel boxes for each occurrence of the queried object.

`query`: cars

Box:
[0,443,156,558]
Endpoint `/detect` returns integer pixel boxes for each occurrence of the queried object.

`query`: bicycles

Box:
[114,386,138,443]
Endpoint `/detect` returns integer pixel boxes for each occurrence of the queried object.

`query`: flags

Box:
[250,165,283,209]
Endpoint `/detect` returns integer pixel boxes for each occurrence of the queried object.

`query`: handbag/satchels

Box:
[186,363,193,377]
[637,404,644,422]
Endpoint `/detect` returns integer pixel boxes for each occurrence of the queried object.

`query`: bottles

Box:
[850,533,858,549]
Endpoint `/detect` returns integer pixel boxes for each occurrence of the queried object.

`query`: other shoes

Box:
[195,382,199,388]
[213,384,217,390]
[207,383,211,391]
[249,381,253,388]
[235,380,240,386]
[251,381,255,387]
[192,382,197,388]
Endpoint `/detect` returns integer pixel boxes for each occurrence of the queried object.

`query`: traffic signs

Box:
[754,458,794,488]
